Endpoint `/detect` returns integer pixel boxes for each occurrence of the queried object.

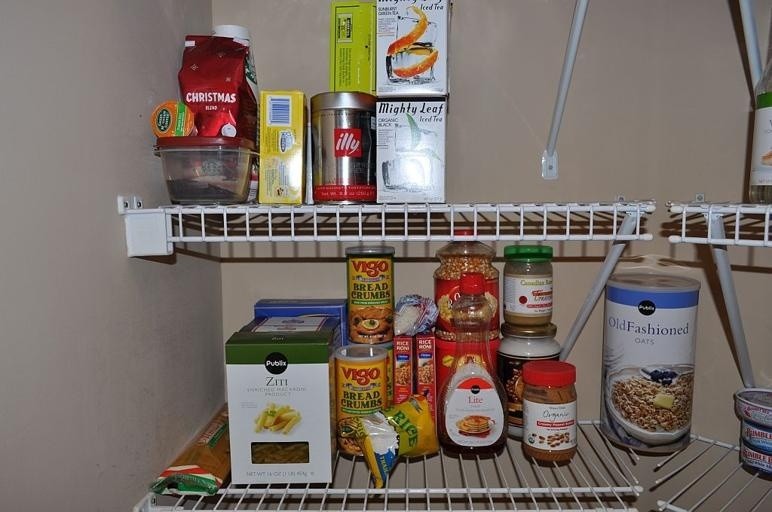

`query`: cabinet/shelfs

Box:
[117,195,655,512]
[653,200,772,512]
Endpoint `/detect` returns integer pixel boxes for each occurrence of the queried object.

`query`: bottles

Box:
[439,273,509,455]
[434,229,499,385]
[741,34,772,204]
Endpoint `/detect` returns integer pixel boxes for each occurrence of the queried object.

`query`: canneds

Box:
[603,271,701,455]
[522,361,576,465]
[504,245,553,325]
[498,324,562,440]
[310,91,379,206]
[345,248,395,345]
[334,346,390,459]
